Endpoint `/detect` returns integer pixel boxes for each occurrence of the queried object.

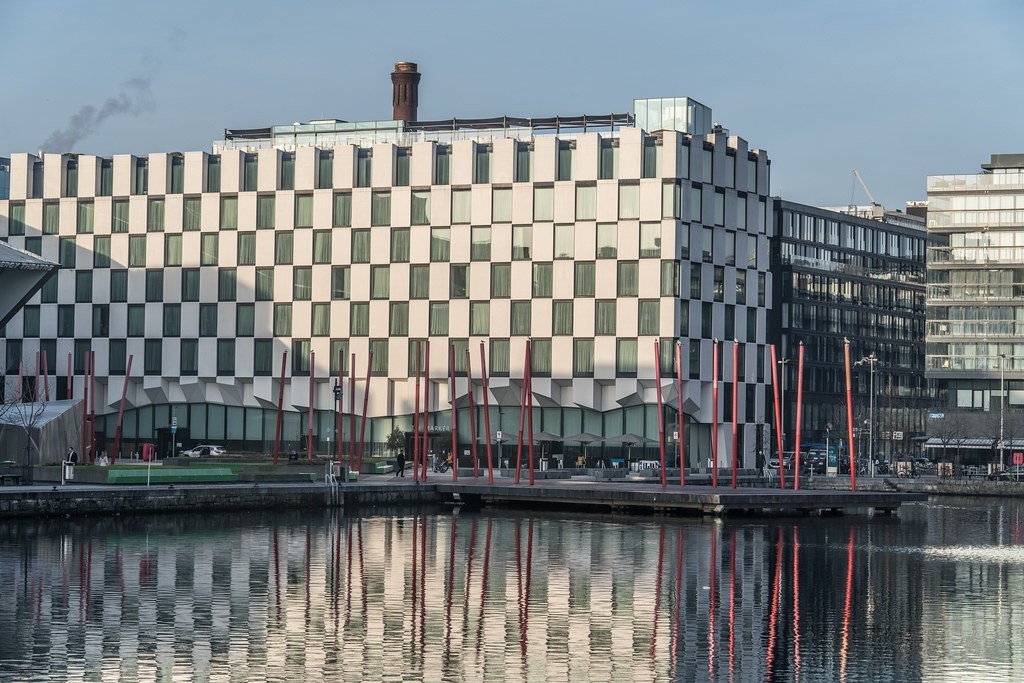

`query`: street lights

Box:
[333,386,341,461]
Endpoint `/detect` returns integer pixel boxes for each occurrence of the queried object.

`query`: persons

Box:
[396,449,405,478]
[98,450,109,467]
[757,451,767,478]
[445,452,454,472]
[67,446,78,466]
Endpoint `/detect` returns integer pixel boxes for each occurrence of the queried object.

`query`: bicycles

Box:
[897,466,920,480]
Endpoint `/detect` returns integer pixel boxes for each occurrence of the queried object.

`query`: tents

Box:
[0,398,83,465]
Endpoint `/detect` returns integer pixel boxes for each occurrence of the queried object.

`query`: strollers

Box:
[433,459,447,473]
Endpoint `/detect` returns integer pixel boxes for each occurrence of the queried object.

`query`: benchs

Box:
[375,461,393,474]
[349,466,359,482]
[0,474,23,486]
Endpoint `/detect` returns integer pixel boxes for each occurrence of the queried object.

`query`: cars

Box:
[915,458,933,468]
[183,445,226,457]
[804,449,827,475]
[769,451,806,469]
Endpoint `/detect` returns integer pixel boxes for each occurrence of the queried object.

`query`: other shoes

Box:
[401,475,404,477]
[396,472,398,477]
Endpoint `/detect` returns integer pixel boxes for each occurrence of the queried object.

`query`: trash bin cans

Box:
[65,465,74,480]
[333,463,341,477]
[540,458,549,471]
[427,455,433,467]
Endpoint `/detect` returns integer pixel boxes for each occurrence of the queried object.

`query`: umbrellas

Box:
[476,429,518,469]
[601,433,661,469]
[556,432,607,469]
[518,431,565,468]
